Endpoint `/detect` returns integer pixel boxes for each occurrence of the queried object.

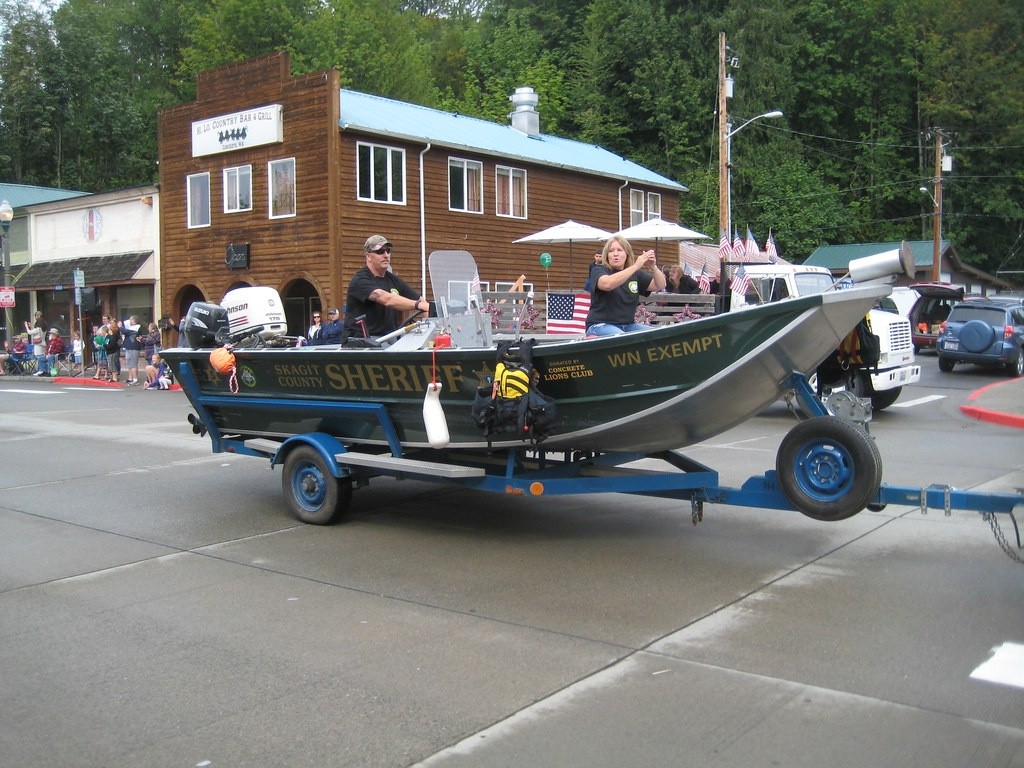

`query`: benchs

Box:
[473,292,717,342]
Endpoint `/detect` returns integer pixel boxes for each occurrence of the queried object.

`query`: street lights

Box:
[919,187,940,283]
[0,201,14,352]
[719,111,784,246]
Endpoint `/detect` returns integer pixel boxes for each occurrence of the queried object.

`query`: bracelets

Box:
[415,299,421,308]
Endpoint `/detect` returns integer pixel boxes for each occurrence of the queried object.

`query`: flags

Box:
[730,263,752,295]
[717,229,777,260]
[547,292,591,335]
[698,263,710,295]
[471,267,481,294]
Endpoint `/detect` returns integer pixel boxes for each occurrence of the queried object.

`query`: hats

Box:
[328,308,339,314]
[47,327,60,335]
[362,235,394,253]
[12,334,21,338]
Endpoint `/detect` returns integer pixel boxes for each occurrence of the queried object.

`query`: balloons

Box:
[540,252,553,267]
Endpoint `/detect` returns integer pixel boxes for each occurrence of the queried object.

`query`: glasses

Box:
[313,316,320,318]
[368,248,393,255]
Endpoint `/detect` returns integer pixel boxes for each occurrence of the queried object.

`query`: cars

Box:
[889,282,1024,376]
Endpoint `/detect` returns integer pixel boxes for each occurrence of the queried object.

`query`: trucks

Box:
[482,258,922,412]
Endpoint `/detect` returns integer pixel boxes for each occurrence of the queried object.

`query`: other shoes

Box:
[157,386,170,390]
[33,370,43,376]
[93,376,100,380]
[38,371,52,377]
[124,378,139,385]
[108,379,119,383]
[103,377,110,381]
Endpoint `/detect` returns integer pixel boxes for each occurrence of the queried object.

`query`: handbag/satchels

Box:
[33,337,43,345]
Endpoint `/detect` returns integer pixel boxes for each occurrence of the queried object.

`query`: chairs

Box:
[0,346,78,376]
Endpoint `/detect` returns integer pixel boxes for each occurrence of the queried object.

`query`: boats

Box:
[158,286,893,455]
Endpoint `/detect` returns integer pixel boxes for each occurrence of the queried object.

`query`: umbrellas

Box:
[511,218,714,291]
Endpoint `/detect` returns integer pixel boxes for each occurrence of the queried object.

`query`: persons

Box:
[322,308,344,344]
[73,330,85,374]
[661,263,700,296]
[585,236,667,337]
[136,317,179,390]
[589,248,602,275]
[0,334,29,375]
[90,314,140,386]
[307,311,325,345]
[24,311,63,377]
[345,234,431,344]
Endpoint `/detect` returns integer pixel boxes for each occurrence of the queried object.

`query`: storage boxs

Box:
[185,286,287,346]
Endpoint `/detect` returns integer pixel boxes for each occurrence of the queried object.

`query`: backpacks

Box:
[816,314,880,401]
[471,337,562,457]
